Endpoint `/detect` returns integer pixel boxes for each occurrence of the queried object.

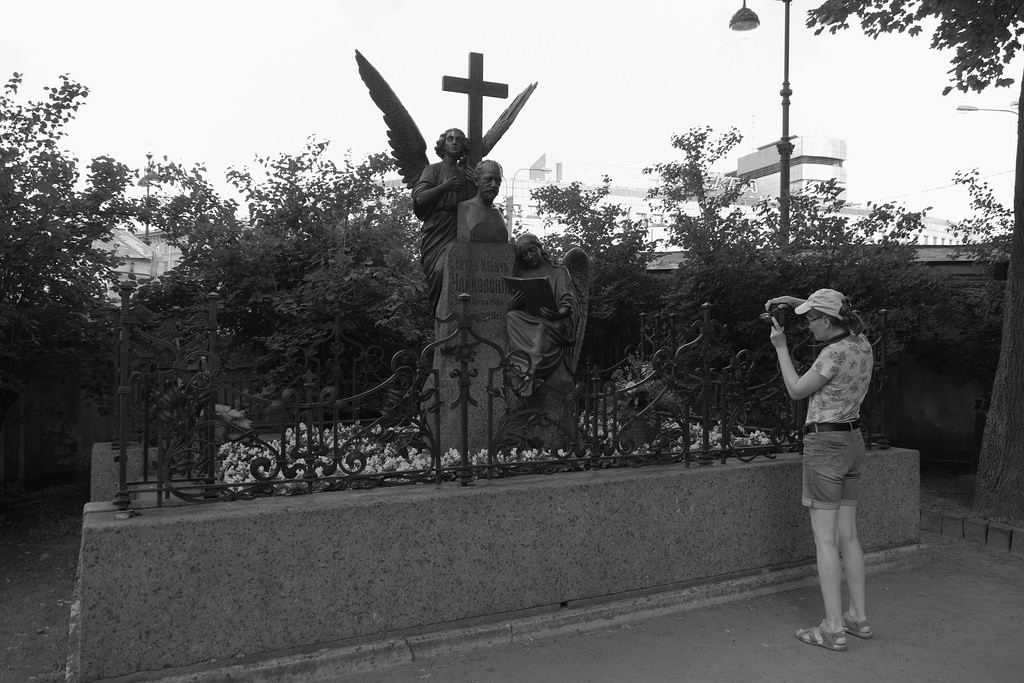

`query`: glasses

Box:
[804,315,836,327]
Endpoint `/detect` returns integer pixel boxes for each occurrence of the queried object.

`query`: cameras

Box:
[759,304,790,328]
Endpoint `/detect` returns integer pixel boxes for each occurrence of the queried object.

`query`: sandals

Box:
[796,619,848,651]
[820,612,873,639]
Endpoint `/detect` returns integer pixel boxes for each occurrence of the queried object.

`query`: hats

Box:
[795,288,846,322]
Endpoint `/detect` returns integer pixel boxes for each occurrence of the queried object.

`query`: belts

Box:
[807,421,860,432]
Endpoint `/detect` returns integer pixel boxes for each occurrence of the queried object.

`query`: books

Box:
[503,276,559,317]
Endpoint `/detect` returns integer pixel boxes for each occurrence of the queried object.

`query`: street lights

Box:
[956,101,1019,117]
[728,2,791,243]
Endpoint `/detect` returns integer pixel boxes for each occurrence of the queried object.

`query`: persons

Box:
[765,289,874,650]
[505,234,578,397]
[457,160,508,242]
[413,128,480,319]
